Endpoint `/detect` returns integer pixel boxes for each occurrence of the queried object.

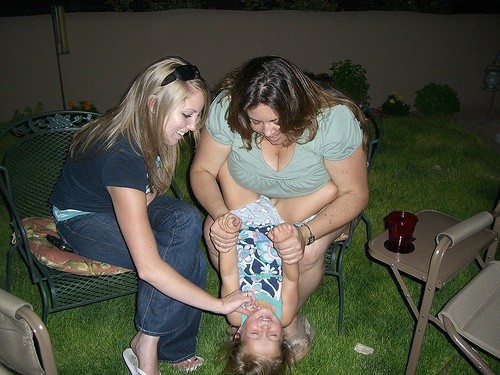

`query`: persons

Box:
[49,56,260,375]
[191,53,369,365]
[218,159,340,375]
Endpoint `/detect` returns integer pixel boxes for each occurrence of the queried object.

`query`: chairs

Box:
[325,107,383,334]
[368,200,500,375]
[0,110,185,326]
[0,289,58,375]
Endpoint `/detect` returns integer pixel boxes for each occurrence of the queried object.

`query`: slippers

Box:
[173,356,205,371]
[287,318,311,363]
[123,347,162,375]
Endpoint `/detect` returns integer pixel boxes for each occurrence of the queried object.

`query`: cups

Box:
[386,210,418,253]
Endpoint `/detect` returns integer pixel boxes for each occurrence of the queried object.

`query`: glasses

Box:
[160,65,201,86]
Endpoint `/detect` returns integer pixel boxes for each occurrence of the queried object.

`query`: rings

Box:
[247,293,248,297]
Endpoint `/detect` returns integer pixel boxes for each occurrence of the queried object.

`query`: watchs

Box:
[305,224,315,245]
